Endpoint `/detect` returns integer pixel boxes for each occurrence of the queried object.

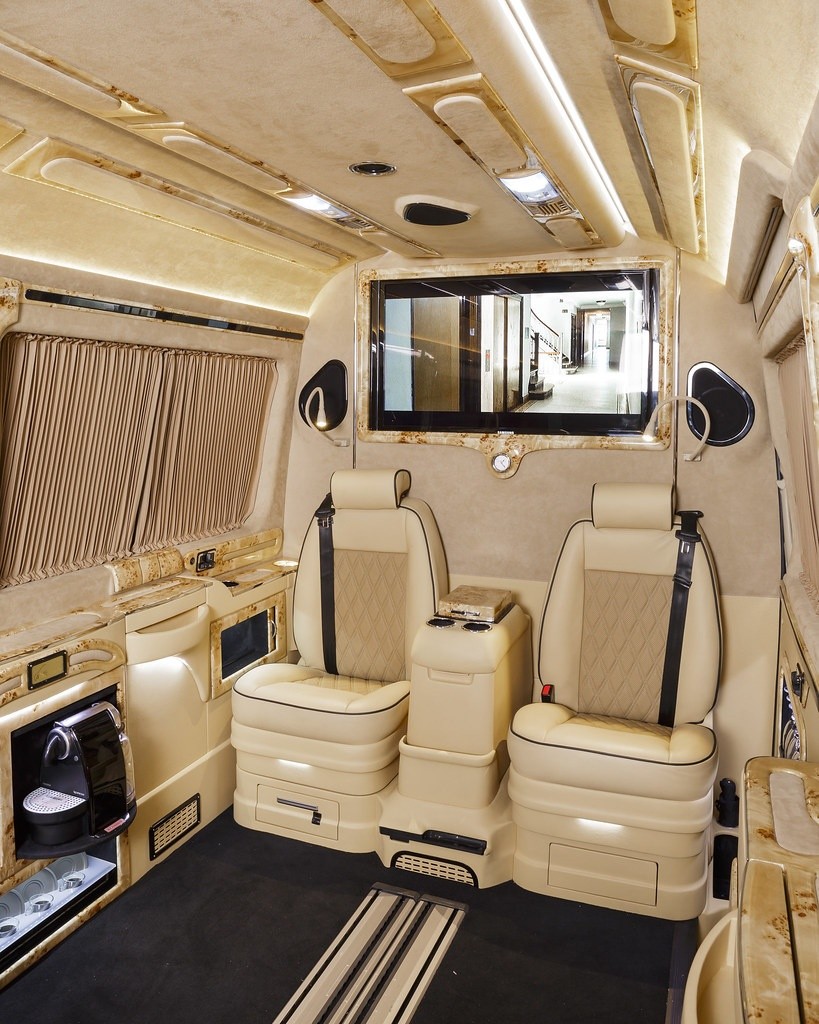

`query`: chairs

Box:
[505,481,725,923]
[229,470,451,854]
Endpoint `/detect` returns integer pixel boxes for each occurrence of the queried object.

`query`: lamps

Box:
[305,387,350,447]
[642,396,710,462]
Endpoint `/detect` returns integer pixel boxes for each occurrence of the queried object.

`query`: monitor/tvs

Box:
[369,268,661,436]
